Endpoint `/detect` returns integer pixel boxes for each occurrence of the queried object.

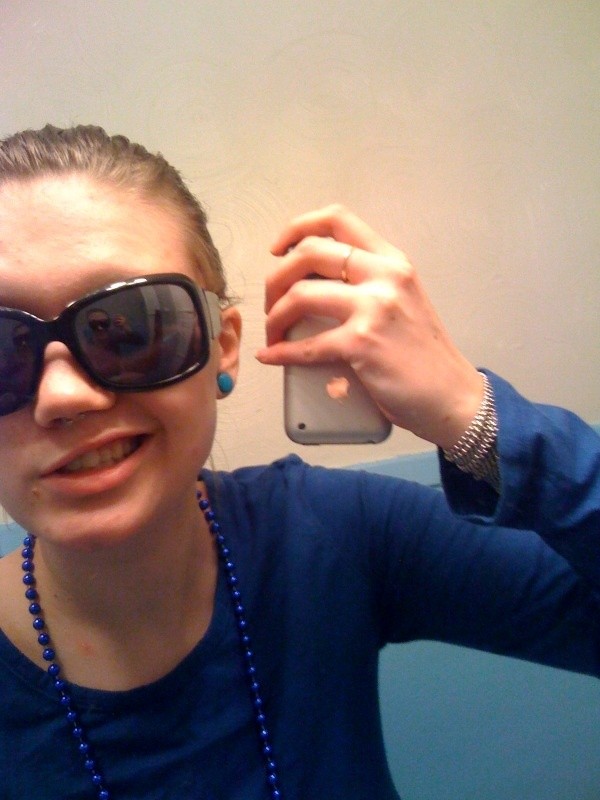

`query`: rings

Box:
[340,245,357,282]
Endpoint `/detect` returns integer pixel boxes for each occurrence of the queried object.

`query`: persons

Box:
[0,125,600,800]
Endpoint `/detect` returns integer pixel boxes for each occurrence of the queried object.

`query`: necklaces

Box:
[21,489,284,800]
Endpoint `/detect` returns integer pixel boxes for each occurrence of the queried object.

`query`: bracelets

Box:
[439,369,503,495]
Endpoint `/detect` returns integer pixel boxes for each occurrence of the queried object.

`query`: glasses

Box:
[0,273,231,416]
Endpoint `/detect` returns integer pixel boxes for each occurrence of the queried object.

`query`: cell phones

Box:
[282,242,394,446]
